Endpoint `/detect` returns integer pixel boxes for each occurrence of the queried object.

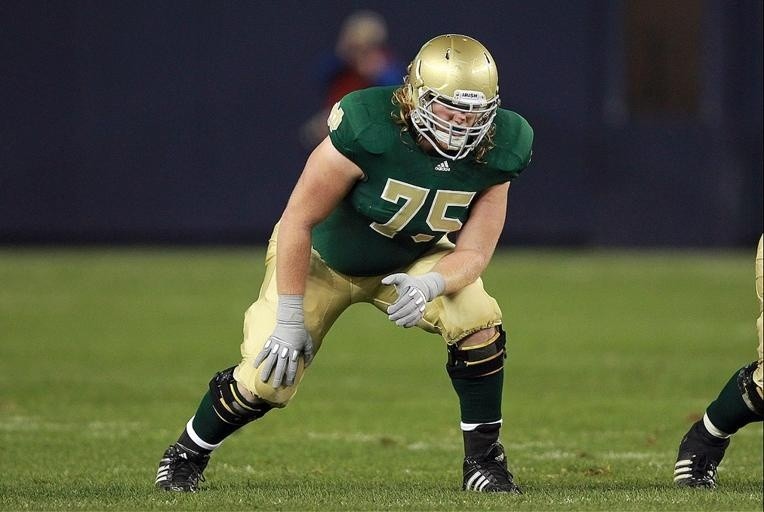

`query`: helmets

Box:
[407,34,500,109]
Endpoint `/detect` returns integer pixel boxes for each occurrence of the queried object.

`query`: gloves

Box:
[253,296,314,388]
[381,271,444,328]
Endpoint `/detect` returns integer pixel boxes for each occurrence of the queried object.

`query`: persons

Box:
[670,226,763,487]
[300,6,414,146]
[153,30,538,497]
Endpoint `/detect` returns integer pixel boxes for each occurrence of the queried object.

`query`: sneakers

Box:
[156,444,211,494]
[674,423,730,488]
[463,444,523,494]
